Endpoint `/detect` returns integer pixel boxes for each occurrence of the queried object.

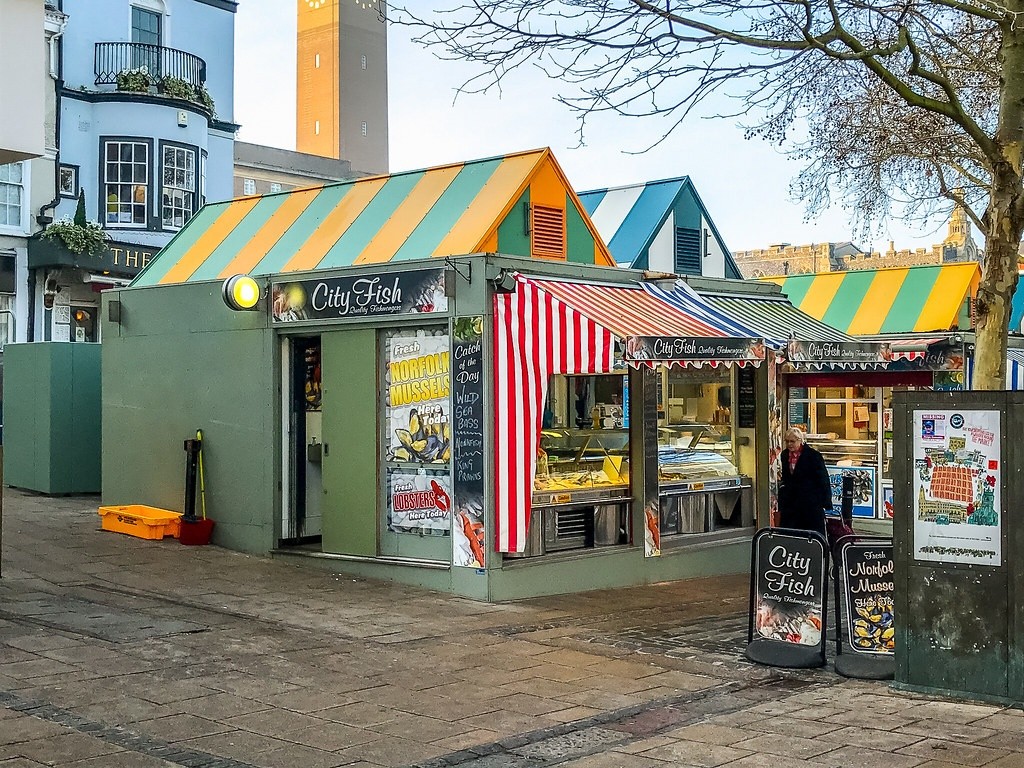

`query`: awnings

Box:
[492,271,764,554]
[861,336,956,361]
[1006,348,1024,390]
[677,279,891,532]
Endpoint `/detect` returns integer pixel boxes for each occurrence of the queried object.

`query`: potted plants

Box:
[195,87,220,124]
[117,72,150,93]
[157,78,194,102]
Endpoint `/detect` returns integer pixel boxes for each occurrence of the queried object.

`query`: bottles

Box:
[725,408,731,425]
[651,404,715,424]
[719,406,724,424]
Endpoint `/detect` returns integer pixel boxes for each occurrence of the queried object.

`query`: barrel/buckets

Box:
[179,516,215,544]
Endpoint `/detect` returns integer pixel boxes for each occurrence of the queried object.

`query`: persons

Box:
[108,188,121,212]
[922,421,934,437]
[777,426,833,541]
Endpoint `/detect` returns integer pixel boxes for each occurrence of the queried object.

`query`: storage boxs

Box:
[99,504,184,540]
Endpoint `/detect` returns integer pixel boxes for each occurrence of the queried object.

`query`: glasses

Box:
[786,437,799,444]
